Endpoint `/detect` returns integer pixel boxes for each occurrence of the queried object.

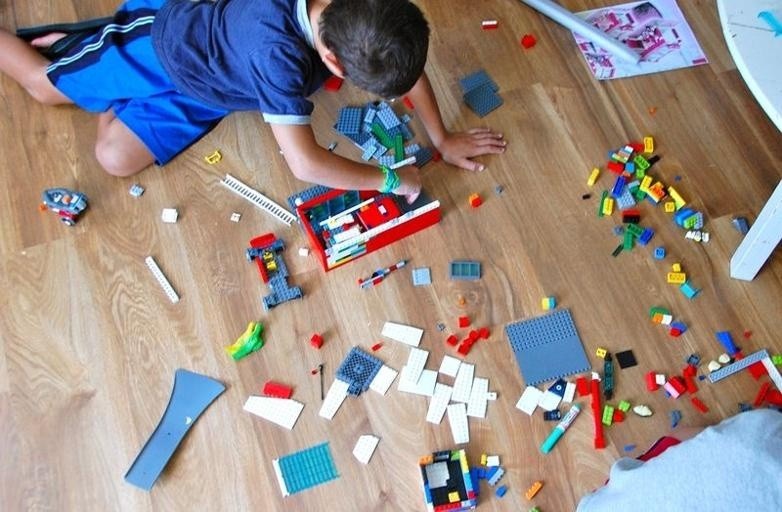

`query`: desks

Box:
[717,0,782,283]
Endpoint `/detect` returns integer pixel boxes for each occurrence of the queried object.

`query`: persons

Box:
[0,0,508,205]
[576,409,782,511]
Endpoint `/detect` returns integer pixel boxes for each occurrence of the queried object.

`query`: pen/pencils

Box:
[540,404,581,455]
[358,261,406,288]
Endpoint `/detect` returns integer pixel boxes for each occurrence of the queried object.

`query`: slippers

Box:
[15,16,116,61]
